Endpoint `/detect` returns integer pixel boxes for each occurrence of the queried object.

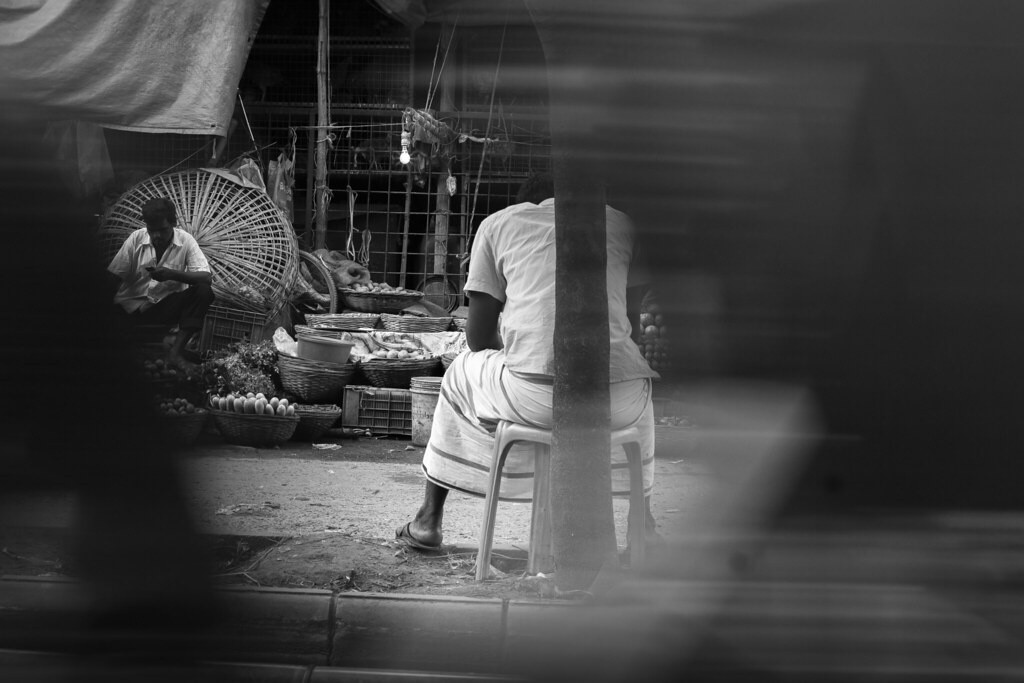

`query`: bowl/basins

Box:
[296,333,355,364]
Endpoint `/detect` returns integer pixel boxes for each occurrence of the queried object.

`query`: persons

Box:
[106,196,217,367]
[394,172,658,557]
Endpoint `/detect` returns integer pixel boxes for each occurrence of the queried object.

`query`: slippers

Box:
[396,522,442,549]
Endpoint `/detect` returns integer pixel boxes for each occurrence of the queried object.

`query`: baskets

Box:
[381,312,454,330]
[357,358,440,387]
[303,313,381,330]
[288,403,342,443]
[138,376,177,396]
[159,410,211,448]
[294,325,342,339]
[452,318,467,330]
[342,291,426,312]
[178,380,231,407]
[208,406,300,446]
[276,351,358,402]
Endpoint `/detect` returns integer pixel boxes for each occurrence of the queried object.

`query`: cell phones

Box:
[145,265,157,274]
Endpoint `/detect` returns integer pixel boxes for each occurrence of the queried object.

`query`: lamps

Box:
[399,131,410,164]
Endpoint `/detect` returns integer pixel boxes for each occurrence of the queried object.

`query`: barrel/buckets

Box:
[409,376,445,446]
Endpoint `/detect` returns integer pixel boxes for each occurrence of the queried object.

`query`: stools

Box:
[476,420,646,585]
